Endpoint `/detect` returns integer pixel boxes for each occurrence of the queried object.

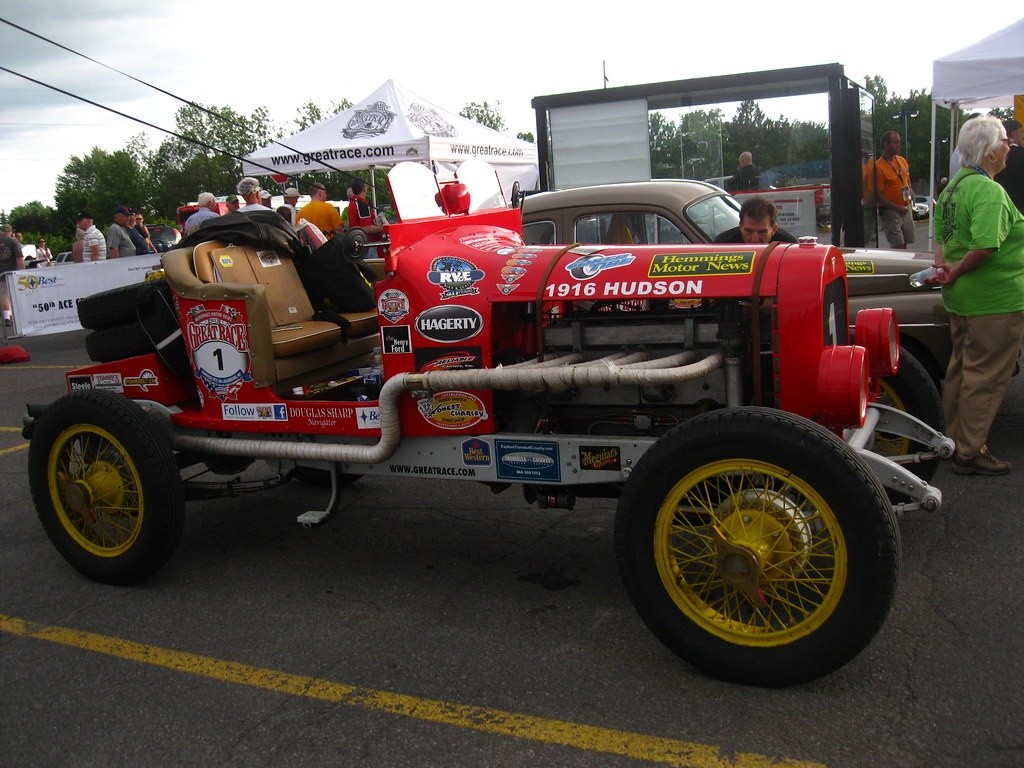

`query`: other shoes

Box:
[5,315,14,325]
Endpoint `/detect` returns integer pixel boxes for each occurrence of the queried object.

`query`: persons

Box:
[73,206,157,263]
[876,131,915,248]
[0,224,53,326]
[733,151,769,190]
[924,113,1024,475]
[713,197,798,243]
[184,177,383,258]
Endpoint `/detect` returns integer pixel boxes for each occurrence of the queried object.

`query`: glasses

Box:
[39,241,45,243]
[1000,138,1011,146]
[135,218,143,220]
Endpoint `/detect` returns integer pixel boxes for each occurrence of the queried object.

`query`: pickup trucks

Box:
[705,175,831,219]
[356,179,956,386]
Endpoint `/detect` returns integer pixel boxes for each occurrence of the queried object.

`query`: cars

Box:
[20,190,956,690]
[911,194,937,220]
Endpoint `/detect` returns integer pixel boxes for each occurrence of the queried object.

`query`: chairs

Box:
[197,241,336,353]
[298,232,388,337]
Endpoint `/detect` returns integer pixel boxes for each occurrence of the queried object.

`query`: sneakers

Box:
[951,443,1012,476]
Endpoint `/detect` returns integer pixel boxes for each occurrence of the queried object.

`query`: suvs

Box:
[54,251,74,265]
[145,223,182,252]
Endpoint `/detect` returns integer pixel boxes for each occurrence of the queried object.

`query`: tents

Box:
[928,17,1024,250]
[242,79,539,205]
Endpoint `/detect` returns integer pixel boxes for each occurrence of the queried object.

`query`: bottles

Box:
[909,266,944,288]
[370,346,384,378]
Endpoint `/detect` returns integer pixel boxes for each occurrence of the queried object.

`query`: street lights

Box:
[929,137,948,182]
[893,110,919,161]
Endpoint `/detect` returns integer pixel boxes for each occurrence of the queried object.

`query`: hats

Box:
[284,188,304,198]
[1002,119,1022,136]
[225,194,239,203]
[1,223,12,233]
[128,208,136,215]
[115,207,134,215]
[259,189,272,200]
[72,210,94,219]
